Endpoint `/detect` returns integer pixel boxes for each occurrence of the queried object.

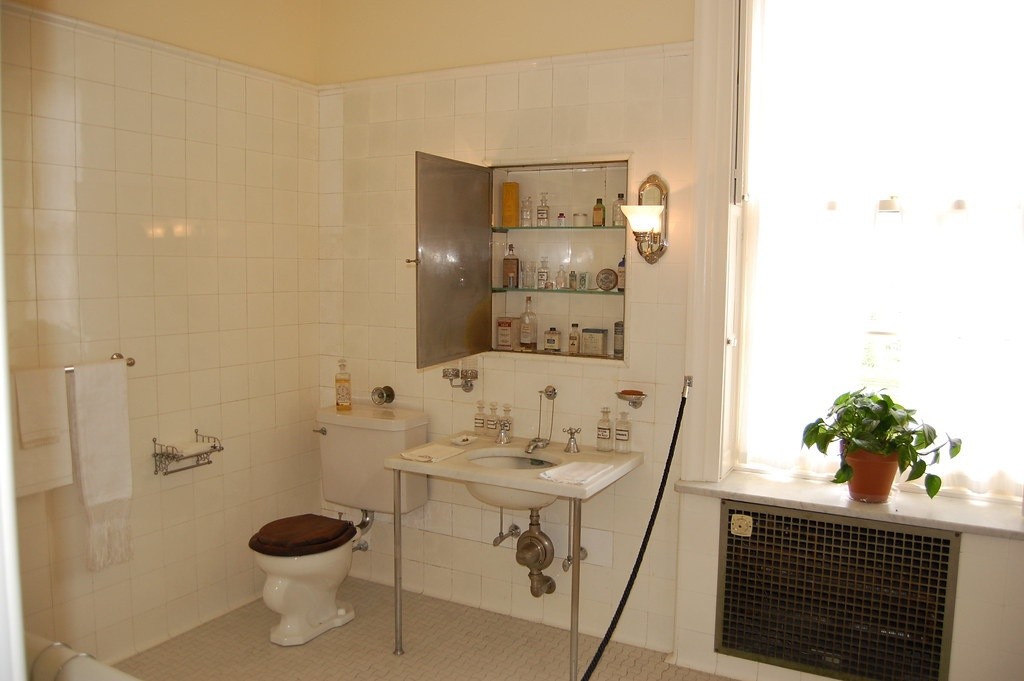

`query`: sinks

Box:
[465,448,563,510]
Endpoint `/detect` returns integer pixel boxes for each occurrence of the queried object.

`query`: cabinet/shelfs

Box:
[406,149,629,369]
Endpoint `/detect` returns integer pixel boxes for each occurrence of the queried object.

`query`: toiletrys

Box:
[597,407,632,454]
[497,181,626,357]
[334,358,351,412]
[473,401,514,437]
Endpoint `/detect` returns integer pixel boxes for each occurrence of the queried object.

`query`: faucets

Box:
[525,437,549,454]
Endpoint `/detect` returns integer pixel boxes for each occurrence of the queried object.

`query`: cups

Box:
[573,213,587,226]
[508,276,515,288]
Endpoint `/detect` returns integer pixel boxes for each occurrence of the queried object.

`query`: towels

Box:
[10,364,70,496]
[165,442,213,458]
[71,360,133,507]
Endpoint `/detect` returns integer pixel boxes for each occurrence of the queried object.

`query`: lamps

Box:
[621,174,669,265]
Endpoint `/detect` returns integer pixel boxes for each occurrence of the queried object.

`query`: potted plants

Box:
[801,387,963,504]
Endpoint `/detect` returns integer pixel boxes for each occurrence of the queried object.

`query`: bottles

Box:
[503,244,519,288]
[521,197,533,227]
[597,408,614,451]
[500,409,513,438]
[569,271,577,290]
[537,260,550,288]
[537,192,550,227]
[569,323,580,354]
[556,269,567,289]
[612,193,626,226]
[488,407,500,437]
[520,296,537,350]
[558,212,565,226]
[614,412,632,454]
[617,255,625,292]
[474,406,487,435]
[593,198,605,226]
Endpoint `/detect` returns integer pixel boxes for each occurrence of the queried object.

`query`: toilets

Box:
[249,403,429,645]
